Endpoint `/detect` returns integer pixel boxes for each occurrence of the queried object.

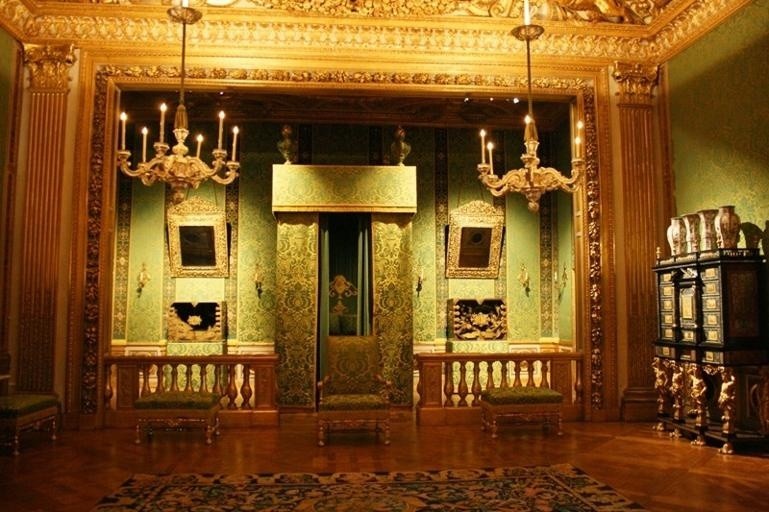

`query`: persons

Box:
[669,360,684,420]
[756,365,769,435]
[687,364,707,426]
[652,357,669,414]
[718,366,736,434]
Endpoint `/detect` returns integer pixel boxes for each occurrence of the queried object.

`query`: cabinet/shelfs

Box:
[647,245,769,455]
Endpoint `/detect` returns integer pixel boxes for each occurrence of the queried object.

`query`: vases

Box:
[666,202,741,258]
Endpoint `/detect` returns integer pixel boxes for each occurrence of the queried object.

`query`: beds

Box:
[266,123,420,413]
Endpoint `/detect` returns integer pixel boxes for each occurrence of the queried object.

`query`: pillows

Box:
[341,313,358,333]
[327,313,340,333]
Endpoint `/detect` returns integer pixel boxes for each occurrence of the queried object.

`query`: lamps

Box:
[476,1,585,214]
[114,1,243,206]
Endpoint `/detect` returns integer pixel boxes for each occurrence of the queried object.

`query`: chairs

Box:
[313,330,394,453]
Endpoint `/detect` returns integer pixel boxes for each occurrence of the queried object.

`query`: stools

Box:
[1,390,60,456]
[133,389,222,444]
[480,386,566,440]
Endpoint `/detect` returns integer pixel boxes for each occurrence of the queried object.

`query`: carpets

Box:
[89,457,650,512]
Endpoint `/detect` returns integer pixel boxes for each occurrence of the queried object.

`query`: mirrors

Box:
[165,193,231,280]
[445,197,506,280]
